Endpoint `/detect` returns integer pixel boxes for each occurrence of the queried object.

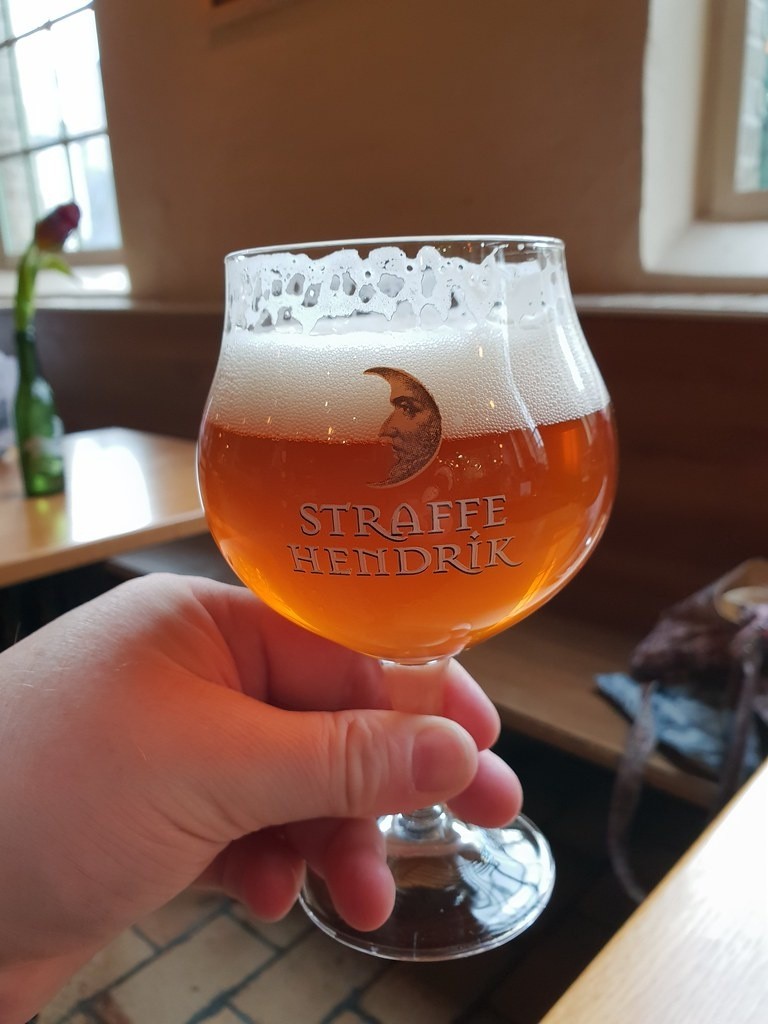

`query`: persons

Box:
[0,571,522,1024]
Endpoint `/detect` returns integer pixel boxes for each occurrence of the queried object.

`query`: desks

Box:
[0,427,212,587]
[534,764,768,1024]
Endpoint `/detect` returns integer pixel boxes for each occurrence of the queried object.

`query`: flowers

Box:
[14,202,82,328]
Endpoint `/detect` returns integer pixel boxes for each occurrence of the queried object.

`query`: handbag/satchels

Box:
[595,555,768,906]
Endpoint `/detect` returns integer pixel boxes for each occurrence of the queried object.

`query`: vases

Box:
[15,327,66,497]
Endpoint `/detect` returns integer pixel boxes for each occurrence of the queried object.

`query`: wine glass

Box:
[196,236,615,962]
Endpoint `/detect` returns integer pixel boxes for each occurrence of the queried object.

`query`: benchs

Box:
[107,534,733,810]
